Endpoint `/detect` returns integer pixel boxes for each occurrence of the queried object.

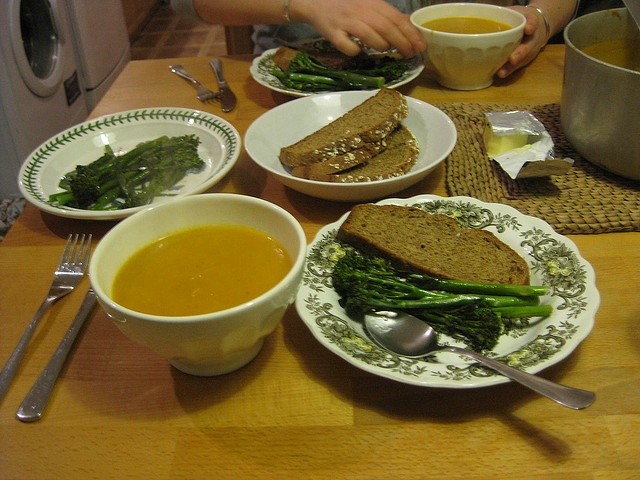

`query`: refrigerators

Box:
[63,0,133,117]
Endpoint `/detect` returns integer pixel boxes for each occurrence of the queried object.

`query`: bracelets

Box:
[281,0,293,25]
[526,5,551,47]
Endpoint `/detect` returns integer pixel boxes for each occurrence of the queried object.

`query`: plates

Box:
[247,36,427,97]
[295,193,601,389]
[19,107,241,221]
[242,91,459,206]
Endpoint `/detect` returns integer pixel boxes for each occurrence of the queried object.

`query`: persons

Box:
[193,0,578,79]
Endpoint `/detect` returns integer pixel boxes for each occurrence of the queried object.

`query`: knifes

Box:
[16,286,98,422]
[208,56,236,112]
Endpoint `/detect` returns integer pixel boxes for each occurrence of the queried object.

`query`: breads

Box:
[279,88,419,184]
[336,203,529,288]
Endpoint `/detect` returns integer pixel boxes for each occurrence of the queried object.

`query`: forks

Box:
[1,232,96,401]
[169,64,223,106]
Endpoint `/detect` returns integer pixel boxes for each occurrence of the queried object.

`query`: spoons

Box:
[364,311,597,410]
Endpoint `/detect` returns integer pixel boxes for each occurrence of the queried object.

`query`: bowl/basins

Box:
[88,193,306,378]
[410,3,527,91]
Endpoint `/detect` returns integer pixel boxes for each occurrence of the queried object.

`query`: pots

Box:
[557,5,640,184]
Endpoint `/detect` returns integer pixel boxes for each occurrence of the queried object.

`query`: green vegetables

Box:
[47,134,205,208]
[333,247,552,351]
[268,46,408,90]
